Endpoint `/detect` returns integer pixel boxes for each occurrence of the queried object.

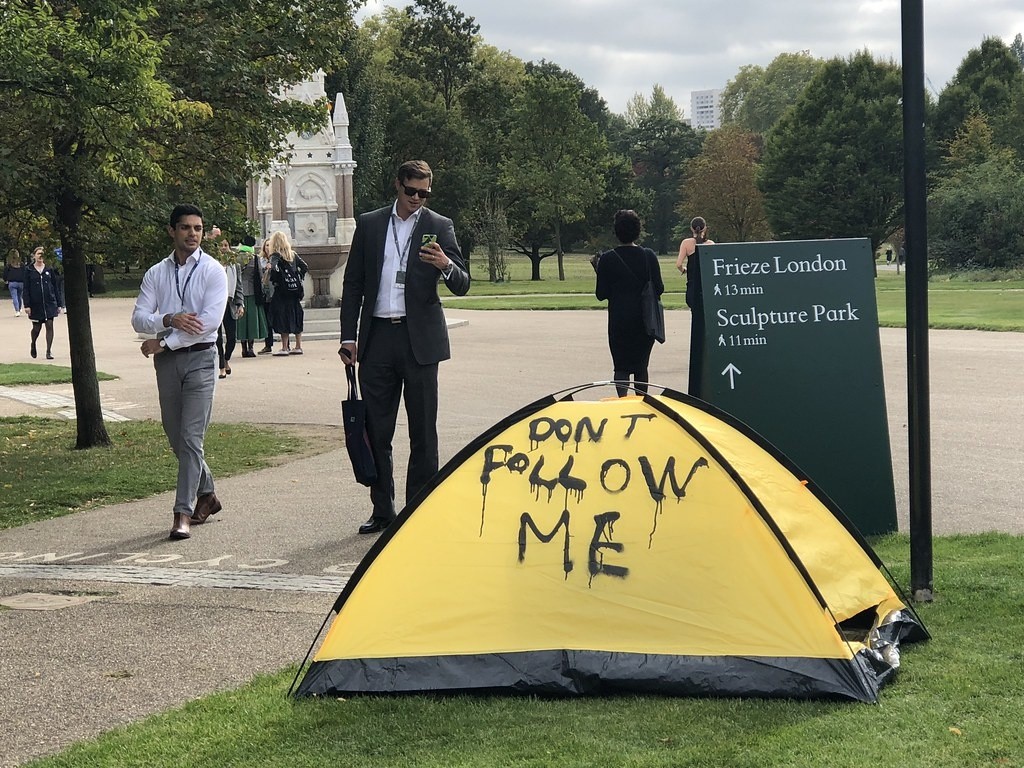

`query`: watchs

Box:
[160,337,170,352]
[443,259,453,274]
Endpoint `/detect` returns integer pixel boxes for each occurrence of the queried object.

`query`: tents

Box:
[284,381,932,714]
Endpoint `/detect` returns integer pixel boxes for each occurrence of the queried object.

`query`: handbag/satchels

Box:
[341,363,378,487]
[253,254,274,304]
[639,248,666,345]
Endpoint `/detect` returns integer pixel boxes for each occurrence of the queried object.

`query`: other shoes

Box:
[289,348,303,355]
[258,348,272,354]
[225,368,232,375]
[272,348,289,356]
[14,312,21,317]
[242,352,249,357]
[30,343,37,358]
[219,373,226,379]
[46,353,55,359]
[249,351,256,357]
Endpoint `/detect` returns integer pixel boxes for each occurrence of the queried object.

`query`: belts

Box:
[169,341,216,353]
[371,316,408,325]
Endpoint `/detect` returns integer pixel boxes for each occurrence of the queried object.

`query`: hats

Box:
[241,235,256,247]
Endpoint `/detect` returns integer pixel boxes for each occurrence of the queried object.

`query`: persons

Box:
[886,247,893,265]
[131,204,229,538]
[201,225,309,379]
[676,217,715,311]
[595,210,665,398]
[3,249,23,317]
[24,247,63,359]
[338,161,471,534]
[899,246,904,265]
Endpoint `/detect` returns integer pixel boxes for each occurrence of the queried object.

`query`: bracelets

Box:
[681,269,685,276]
[169,313,176,327]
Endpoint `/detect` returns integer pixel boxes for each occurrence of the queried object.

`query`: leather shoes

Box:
[359,511,397,534]
[190,492,222,525]
[169,512,191,538]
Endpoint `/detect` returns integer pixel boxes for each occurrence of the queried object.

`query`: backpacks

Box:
[277,256,305,300]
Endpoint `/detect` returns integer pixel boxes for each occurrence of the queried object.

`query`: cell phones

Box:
[419,234,437,259]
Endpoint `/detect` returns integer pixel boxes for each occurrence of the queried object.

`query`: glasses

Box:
[398,180,432,198]
